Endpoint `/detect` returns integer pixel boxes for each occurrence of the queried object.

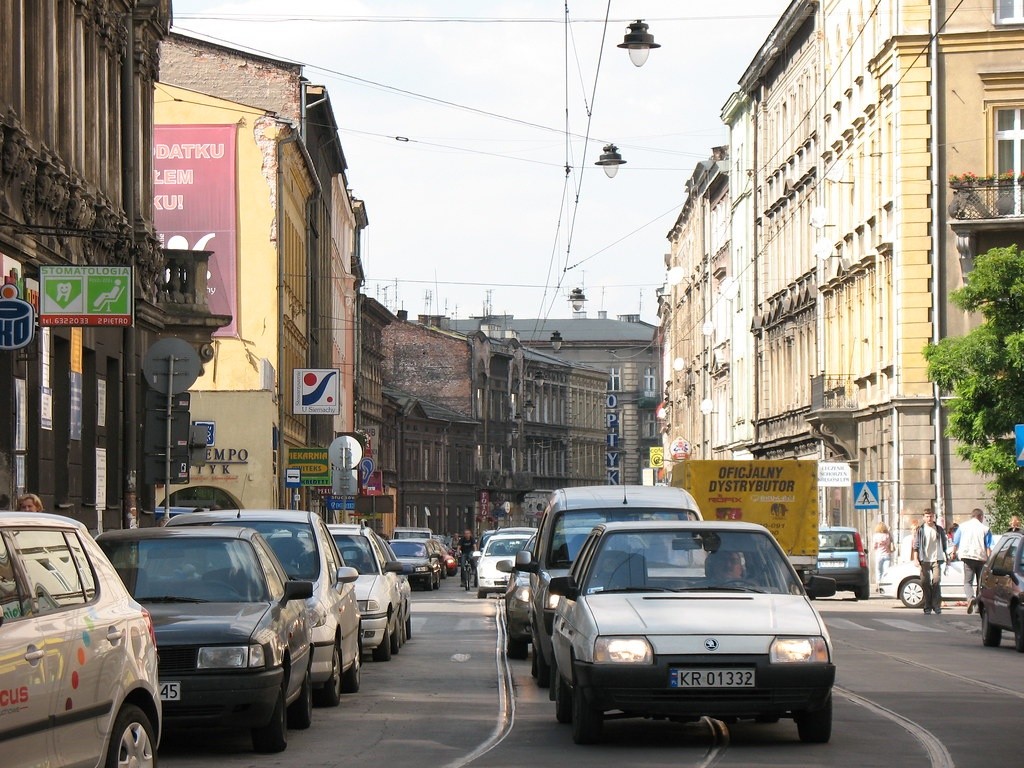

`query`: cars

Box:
[802,525,870,601]
[89,520,315,756]
[878,534,1002,610]
[550,516,838,747]
[136,505,365,711]
[256,520,543,665]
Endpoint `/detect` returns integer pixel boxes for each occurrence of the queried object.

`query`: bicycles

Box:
[457,550,473,591]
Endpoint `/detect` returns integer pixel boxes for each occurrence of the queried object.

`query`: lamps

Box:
[617,21,661,67]
[548,331,564,350]
[511,425,520,439]
[566,288,587,311]
[594,144,626,178]
[532,371,546,386]
[523,400,535,413]
[515,412,522,424]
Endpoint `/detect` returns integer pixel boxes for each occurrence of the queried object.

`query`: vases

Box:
[950,182,973,187]
[998,179,1012,185]
[1020,180,1024,186]
[977,180,993,185]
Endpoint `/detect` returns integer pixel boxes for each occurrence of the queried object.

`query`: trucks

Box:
[667,460,821,596]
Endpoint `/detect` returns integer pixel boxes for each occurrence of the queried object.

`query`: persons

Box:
[951,508,991,614]
[873,521,895,594]
[710,552,742,581]
[914,508,949,616]
[194,504,221,512]
[450,533,461,563]
[0,494,10,510]
[1006,512,1024,535]
[457,529,477,587]
[158,517,169,527]
[911,518,919,567]
[17,493,43,512]
[946,522,959,562]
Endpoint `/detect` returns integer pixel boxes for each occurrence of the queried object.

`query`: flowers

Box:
[948,169,1024,182]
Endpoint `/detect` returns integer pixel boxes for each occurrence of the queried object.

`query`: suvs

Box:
[0,507,166,768]
[972,524,1024,655]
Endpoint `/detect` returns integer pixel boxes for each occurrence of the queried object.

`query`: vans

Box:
[516,483,708,691]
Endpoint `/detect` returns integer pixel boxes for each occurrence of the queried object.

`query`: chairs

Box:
[202,569,247,592]
[593,551,648,586]
[839,535,850,547]
[554,534,588,562]
[116,568,150,594]
[268,539,305,560]
[340,546,373,574]
[494,546,507,555]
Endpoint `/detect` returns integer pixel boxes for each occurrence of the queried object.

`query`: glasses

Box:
[717,557,739,565]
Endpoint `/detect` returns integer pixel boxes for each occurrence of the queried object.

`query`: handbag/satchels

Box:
[889,533,895,552]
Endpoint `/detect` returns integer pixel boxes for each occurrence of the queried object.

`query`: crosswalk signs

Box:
[854,482,881,510]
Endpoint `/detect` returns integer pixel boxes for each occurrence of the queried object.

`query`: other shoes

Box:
[925,608,932,614]
[967,597,975,614]
[933,607,941,614]
